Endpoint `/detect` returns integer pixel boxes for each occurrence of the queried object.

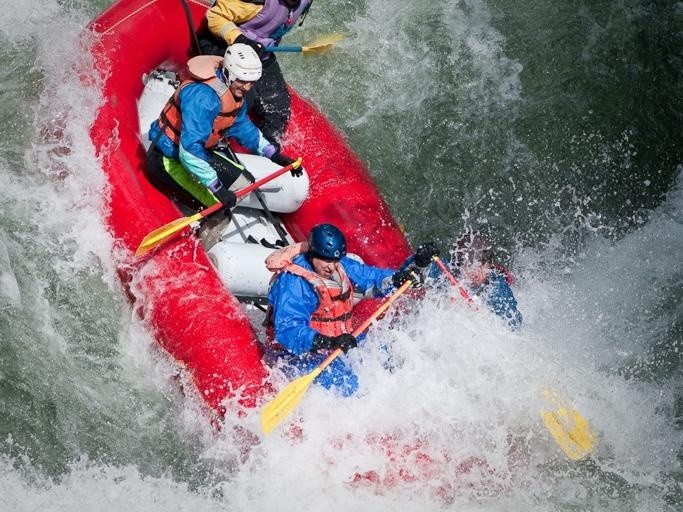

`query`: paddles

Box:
[431,255,599,460]
[135,157,302,258]
[261,281,411,435]
[266,32,346,58]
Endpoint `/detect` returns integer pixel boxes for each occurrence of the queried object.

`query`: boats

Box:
[36,0,490,504]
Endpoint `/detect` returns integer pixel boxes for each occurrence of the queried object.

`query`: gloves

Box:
[393,268,424,288]
[209,179,236,208]
[311,333,357,354]
[271,153,303,177]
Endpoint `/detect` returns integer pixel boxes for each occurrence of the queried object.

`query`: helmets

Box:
[223,43,262,81]
[309,223,346,258]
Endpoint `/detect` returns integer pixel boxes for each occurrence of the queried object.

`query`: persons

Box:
[254,222,423,401]
[194,0,316,142]
[387,230,525,333]
[141,41,305,257]
